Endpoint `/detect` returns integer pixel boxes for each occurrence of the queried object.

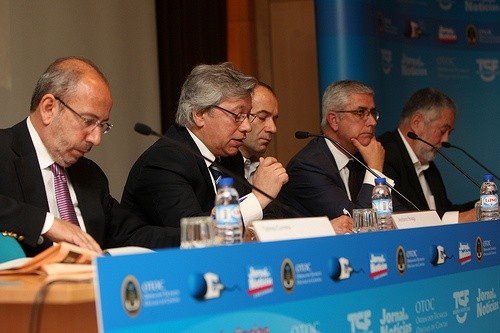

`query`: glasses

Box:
[54,96,114,135]
[334,107,380,121]
[209,104,257,123]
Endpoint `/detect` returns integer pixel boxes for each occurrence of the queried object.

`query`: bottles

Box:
[480,174,500,220]
[372,177,394,232]
[215,177,243,246]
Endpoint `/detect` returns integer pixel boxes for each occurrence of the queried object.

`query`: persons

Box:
[351,88,479,224]
[241,82,353,235]
[280,80,386,221]
[123,62,289,227]
[0,55,181,257]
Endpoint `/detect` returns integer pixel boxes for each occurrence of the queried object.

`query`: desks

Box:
[0,209,500,333]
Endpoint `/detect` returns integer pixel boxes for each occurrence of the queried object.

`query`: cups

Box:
[180,216,216,249]
[352,208,378,233]
[475,200,481,221]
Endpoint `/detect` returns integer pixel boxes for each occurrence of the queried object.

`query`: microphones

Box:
[295,131,421,211]
[440,142,500,182]
[407,131,481,190]
[134,123,303,218]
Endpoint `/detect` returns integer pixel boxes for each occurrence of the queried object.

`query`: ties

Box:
[48,162,81,228]
[345,160,362,203]
[209,157,225,194]
